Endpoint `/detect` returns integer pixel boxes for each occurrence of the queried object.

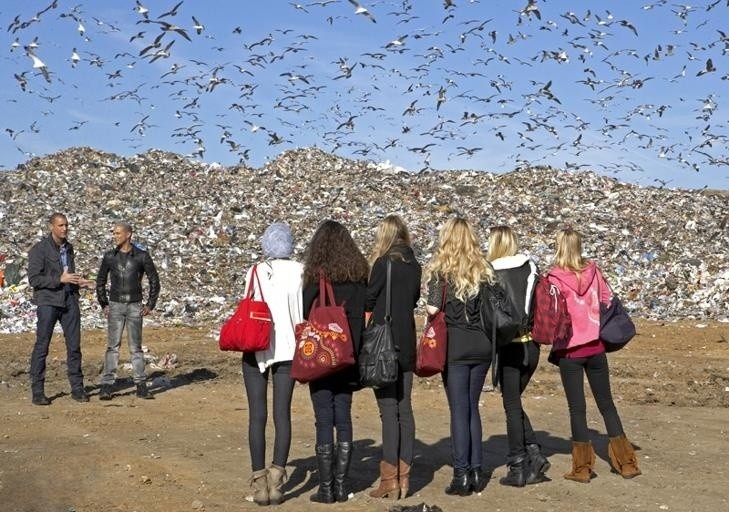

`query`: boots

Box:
[308,442,335,503]
[607,433,641,478]
[524,444,550,484]
[369,460,399,500]
[397,457,414,498]
[246,469,269,505]
[564,439,595,483]
[333,442,352,502]
[266,462,287,506]
[499,452,525,486]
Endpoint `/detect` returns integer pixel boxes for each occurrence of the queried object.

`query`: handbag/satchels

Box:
[413,277,449,377]
[354,256,400,391]
[289,268,355,384]
[599,277,636,352]
[479,281,520,387]
[532,274,573,345]
[218,262,273,352]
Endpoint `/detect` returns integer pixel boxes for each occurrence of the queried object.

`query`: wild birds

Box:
[0,0,729,317]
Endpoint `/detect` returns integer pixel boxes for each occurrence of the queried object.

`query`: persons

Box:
[423,217,495,495]
[97,224,160,399]
[365,215,421,499]
[485,225,550,487]
[303,220,370,503]
[547,229,641,482]
[241,223,305,505]
[27,212,97,405]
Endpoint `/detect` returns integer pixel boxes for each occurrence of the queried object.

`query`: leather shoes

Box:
[32,391,51,405]
[445,474,470,496]
[470,471,486,493]
[71,389,88,402]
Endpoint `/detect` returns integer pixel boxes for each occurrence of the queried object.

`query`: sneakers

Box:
[99,383,113,398]
[137,383,154,399]
